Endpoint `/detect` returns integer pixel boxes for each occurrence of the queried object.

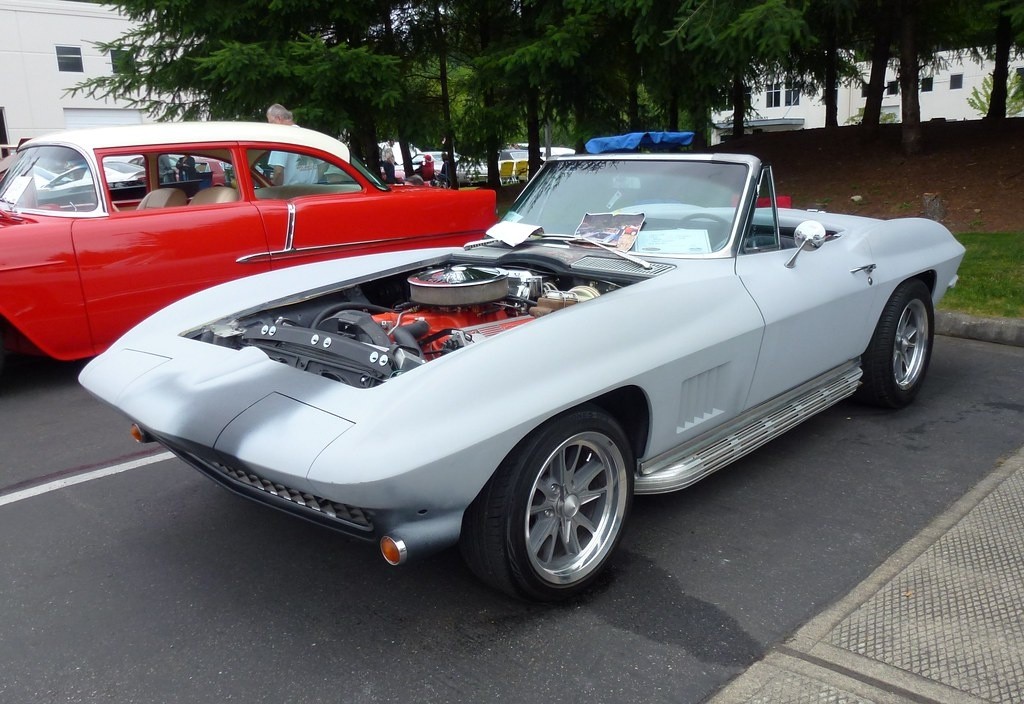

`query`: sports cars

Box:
[74,151,972,603]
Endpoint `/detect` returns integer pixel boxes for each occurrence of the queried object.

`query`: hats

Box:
[423,155,431,159]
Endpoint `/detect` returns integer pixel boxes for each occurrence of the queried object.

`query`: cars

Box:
[370,141,579,184]
[0,120,499,377]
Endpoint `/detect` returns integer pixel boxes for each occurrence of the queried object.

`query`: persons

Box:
[265,103,331,185]
[415,155,434,176]
[174,154,197,182]
[381,138,398,183]
[440,153,450,174]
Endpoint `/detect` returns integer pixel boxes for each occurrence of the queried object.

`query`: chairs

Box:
[515,160,528,183]
[499,162,514,186]
[135,188,187,210]
[187,186,238,207]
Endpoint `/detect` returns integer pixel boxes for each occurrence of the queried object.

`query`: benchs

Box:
[254,184,361,200]
